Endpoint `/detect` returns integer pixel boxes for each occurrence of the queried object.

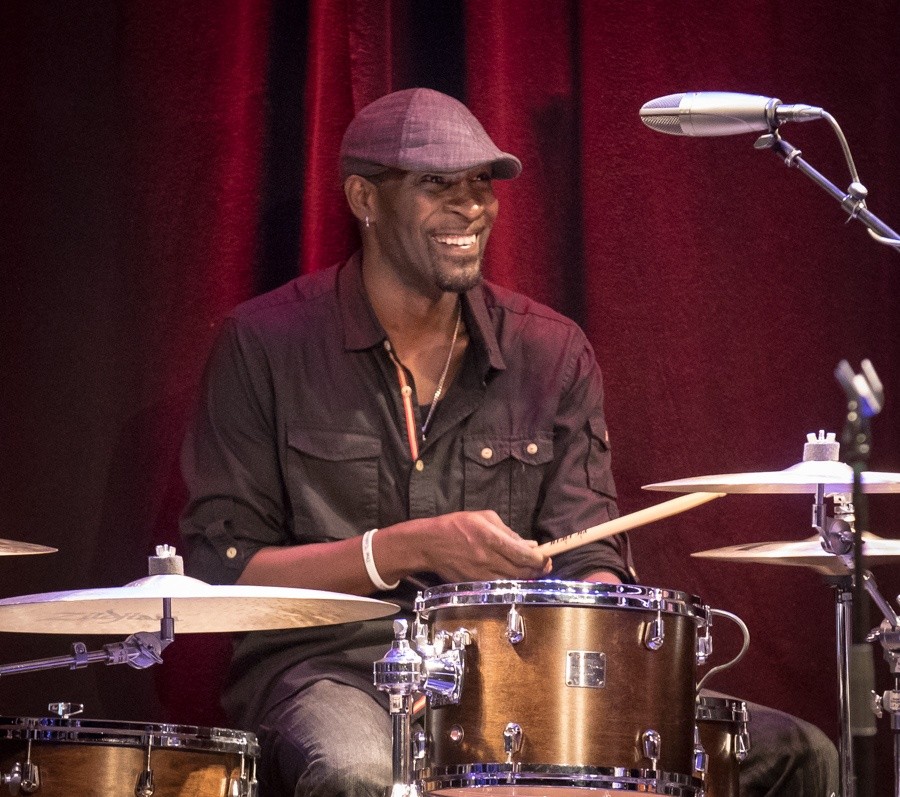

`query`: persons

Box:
[181,87,840,797]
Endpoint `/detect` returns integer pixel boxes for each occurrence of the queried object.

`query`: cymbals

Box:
[641,461,900,496]
[2,571,401,638]
[688,529,900,578]
[1,539,60,559]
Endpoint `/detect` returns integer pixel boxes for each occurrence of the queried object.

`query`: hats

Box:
[340,88,521,180]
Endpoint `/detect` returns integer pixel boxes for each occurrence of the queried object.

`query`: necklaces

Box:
[419,303,463,442]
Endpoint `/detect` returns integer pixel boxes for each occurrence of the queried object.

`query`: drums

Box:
[417,576,710,797]
[1,712,262,797]
[696,694,753,795]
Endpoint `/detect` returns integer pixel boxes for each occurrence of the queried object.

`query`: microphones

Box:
[639,91,824,137]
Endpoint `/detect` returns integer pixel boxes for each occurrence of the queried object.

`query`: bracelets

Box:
[362,529,400,590]
